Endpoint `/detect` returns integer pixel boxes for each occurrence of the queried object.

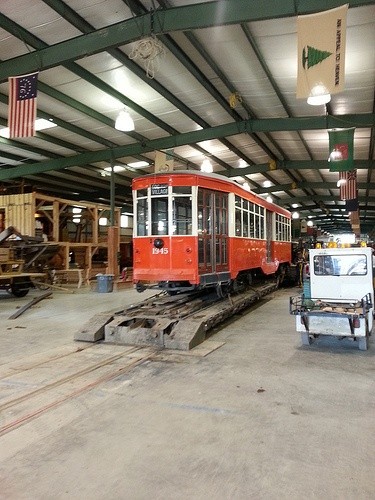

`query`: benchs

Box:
[50,268,90,288]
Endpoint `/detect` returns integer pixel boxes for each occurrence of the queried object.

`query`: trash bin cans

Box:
[96,274,115,293]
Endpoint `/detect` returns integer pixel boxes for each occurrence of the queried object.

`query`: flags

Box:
[8,72,38,138]
[301,221,327,237]
[339,168,357,200]
[346,190,360,238]
[154,148,174,173]
[328,127,355,172]
[296,3,349,99]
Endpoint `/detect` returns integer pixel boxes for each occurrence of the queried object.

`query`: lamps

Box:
[306,80,331,106]
[114,105,135,132]
[201,156,213,173]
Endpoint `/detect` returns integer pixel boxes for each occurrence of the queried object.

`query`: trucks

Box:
[288,241,375,352]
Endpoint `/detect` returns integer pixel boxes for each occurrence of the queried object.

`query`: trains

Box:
[130,163,294,300]
